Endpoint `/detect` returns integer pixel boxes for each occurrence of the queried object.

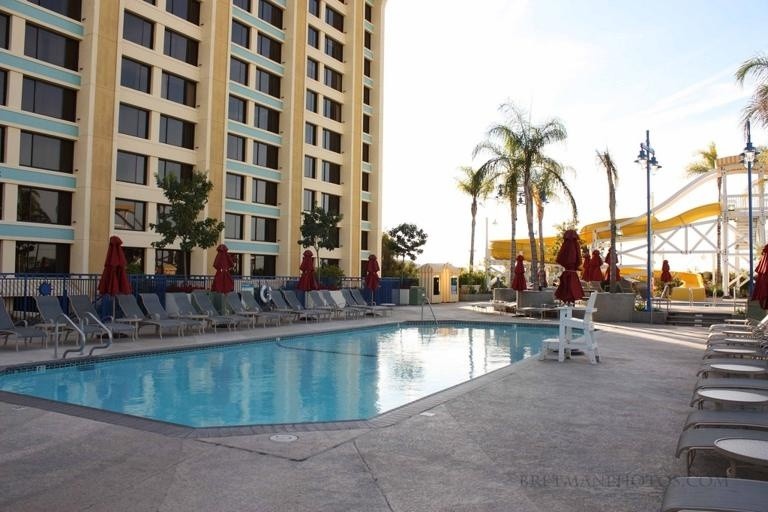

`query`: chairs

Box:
[494,285,672,319]
[660,306,768,511]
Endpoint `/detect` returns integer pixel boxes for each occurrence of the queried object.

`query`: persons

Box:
[537,264,547,290]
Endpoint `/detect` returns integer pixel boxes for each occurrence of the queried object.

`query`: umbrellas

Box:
[751,244,768,310]
[366,255,380,306]
[295,250,319,308]
[97,235,134,323]
[211,244,235,304]
[661,260,672,282]
[554,229,585,306]
[581,247,621,282]
[512,255,527,307]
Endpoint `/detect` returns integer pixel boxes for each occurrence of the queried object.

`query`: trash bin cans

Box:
[409,286,426,305]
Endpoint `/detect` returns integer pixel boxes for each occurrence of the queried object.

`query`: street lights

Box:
[739,116,760,301]
[630,128,662,314]
[483,216,499,281]
